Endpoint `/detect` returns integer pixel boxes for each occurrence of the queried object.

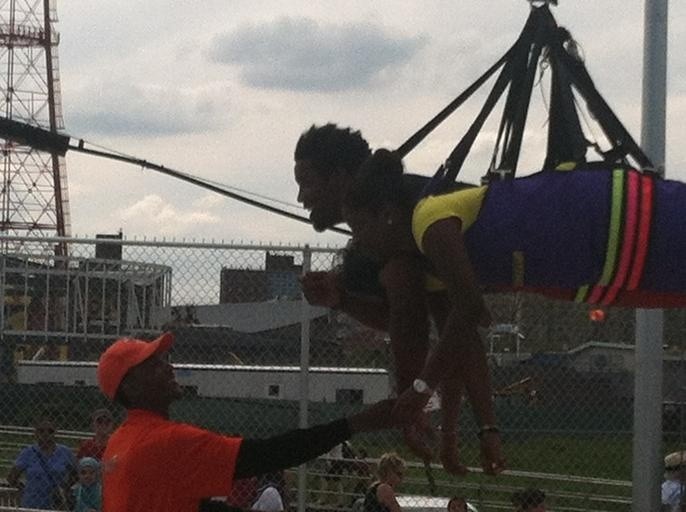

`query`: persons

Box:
[74,455,103,511]
[292,123,517,327]
[447,495,468,511]
[340,145,686,480]
[328,227,511,479]
[657,449,686,511]
[246,470,289,512]
[90,328,398,512]
[72,407,115,463]
[360,452,405,511]
[511,486,547,511]
[4,421,76,510]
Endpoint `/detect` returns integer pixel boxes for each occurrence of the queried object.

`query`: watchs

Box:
[413,378,434,397]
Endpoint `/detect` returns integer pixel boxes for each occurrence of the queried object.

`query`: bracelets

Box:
[477,423,500,439]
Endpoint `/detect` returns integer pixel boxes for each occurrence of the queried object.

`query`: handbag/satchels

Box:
[48,486,68,510]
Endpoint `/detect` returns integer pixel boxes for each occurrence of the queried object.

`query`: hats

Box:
[99,332,173,402]
[662,452,686,479]
[91,408,112,422]
